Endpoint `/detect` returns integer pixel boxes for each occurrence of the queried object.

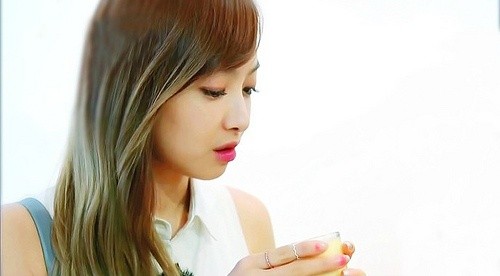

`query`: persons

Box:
[0,0,366,276]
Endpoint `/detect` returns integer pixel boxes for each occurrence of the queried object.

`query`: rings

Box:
[265,251,273,268]
[293,243,300,260]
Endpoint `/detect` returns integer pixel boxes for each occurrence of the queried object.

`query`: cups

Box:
[307,232,347,276]
